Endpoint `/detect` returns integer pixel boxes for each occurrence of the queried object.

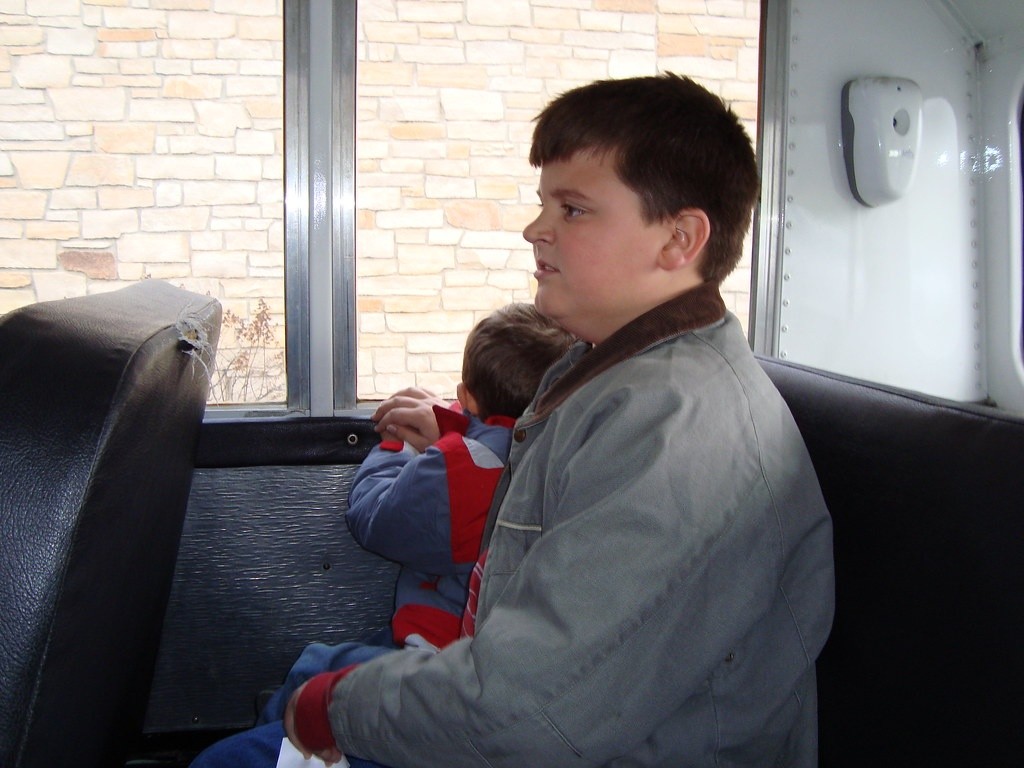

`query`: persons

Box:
[267,302,594,721]
[194,69,837,768]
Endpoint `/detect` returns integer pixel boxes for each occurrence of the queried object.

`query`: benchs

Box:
[754,353,1024,768]
[0,280,220,768]
